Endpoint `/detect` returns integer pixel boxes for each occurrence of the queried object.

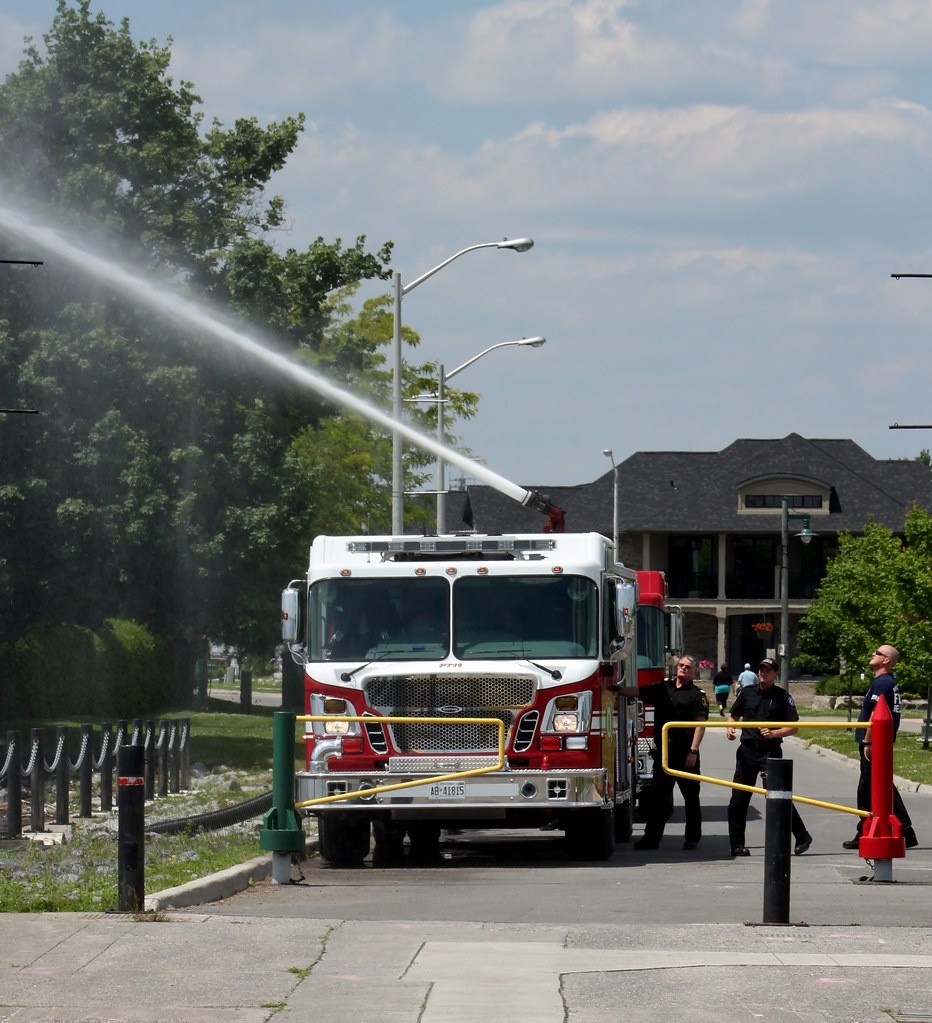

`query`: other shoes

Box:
[730,844,751,856]
[634,839,660,850]
[683,839,697,849]
[842,833,861,849]
[720,709,725,717]
[902,827,918,848]
[794,831,813,854]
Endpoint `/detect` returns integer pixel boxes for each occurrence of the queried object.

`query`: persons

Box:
[736,663,759,699]
[727,658,813,857]
[842,644,918,851]
[712,664,735,716]
[618,655,709,850]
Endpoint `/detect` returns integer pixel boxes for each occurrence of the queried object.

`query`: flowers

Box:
[698,657,716,670]
[752,622,775,633]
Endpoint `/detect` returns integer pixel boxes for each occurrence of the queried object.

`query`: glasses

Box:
[876,651,888,657]
[677,662,692,669]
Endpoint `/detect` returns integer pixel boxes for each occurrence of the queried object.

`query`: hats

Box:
[759,658,780,672]
[744,663,751,669]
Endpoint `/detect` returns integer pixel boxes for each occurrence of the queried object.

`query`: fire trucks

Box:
[279,490,643,859]
[627,569,688,793]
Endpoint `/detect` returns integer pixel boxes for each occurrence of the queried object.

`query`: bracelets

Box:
[861,740,871,745]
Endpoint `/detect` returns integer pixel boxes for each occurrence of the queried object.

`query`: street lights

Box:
[434,334,548,531]
[390,237,535,536]
[604,449,622,564]
[779,498,817,691]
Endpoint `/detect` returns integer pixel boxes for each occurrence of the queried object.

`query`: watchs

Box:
[690,748,699,754]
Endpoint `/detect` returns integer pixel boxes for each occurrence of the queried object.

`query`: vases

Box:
[698,669,712,680]
